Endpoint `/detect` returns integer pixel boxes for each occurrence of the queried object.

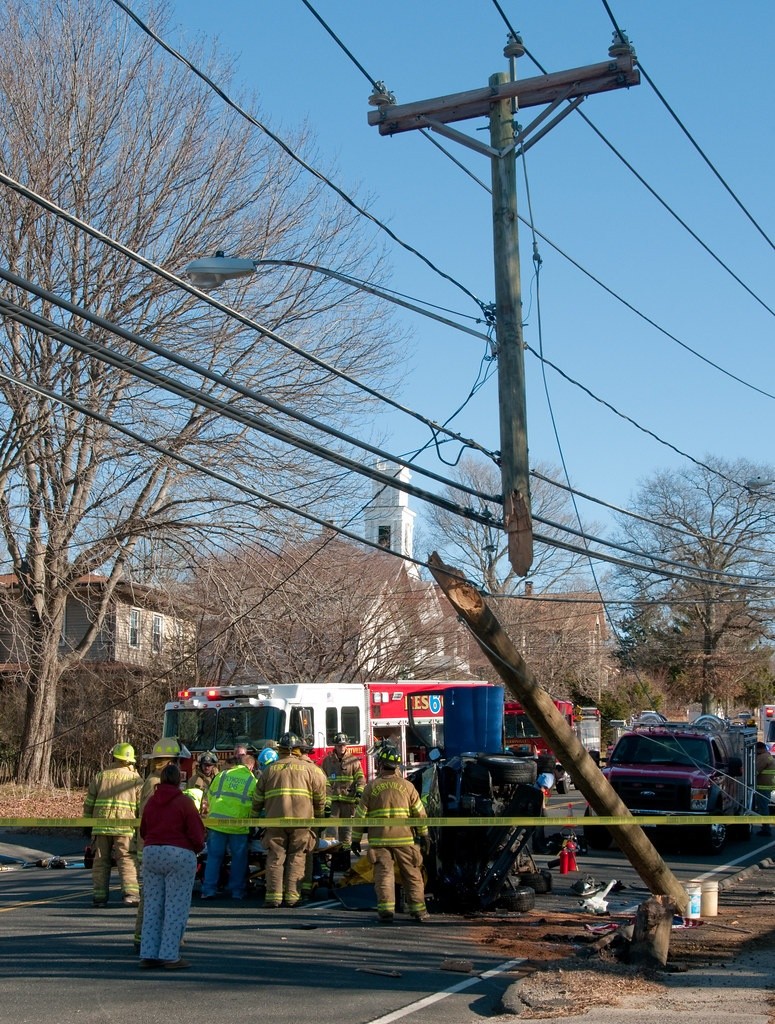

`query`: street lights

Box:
[185,246,542,576]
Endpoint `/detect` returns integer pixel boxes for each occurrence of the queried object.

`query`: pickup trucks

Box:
[580,714,760,854]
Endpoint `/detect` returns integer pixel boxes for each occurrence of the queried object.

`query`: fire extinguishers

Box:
[565,834,581,870]
[560,845,570,873]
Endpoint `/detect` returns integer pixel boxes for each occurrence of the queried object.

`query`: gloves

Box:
[85,827,93,839]
[355,791,362,803]
[351,842,363,857]
[420,836,433,855]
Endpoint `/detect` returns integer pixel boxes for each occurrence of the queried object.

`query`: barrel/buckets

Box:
[677,880,702,920]
[690,880,719,917]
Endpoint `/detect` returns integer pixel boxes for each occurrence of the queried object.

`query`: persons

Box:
[82,743,144,906]
[322,734,366,852]
[139,765,205,969]
[532,766,565,855]
[351,746,431,919]
[539,751,555,774]
[755,741,775,837]
[134,739,186,948]
[184,734,332,908]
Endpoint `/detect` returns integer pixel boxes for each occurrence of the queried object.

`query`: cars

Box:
[403,752,557,914]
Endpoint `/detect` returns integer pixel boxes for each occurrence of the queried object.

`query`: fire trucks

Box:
[763,705,775,756]
[503,700,607,793]
[164,677,509,792]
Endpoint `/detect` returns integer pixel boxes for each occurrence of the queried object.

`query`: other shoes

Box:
[381,914,393,926]
[261,900,278,908]
[201,892,212,899]
[416,910,431,921]
[288,900,304,908]
[164,958,192,969]
[138,958,162,969]
[89,897,107,906]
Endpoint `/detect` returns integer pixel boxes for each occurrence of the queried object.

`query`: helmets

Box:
[278,734,301,750]
[150,738,180,758]
[332,734,349,745]
[374,746,401,762]
[197,753,218,765]
[109,744,136,763]
